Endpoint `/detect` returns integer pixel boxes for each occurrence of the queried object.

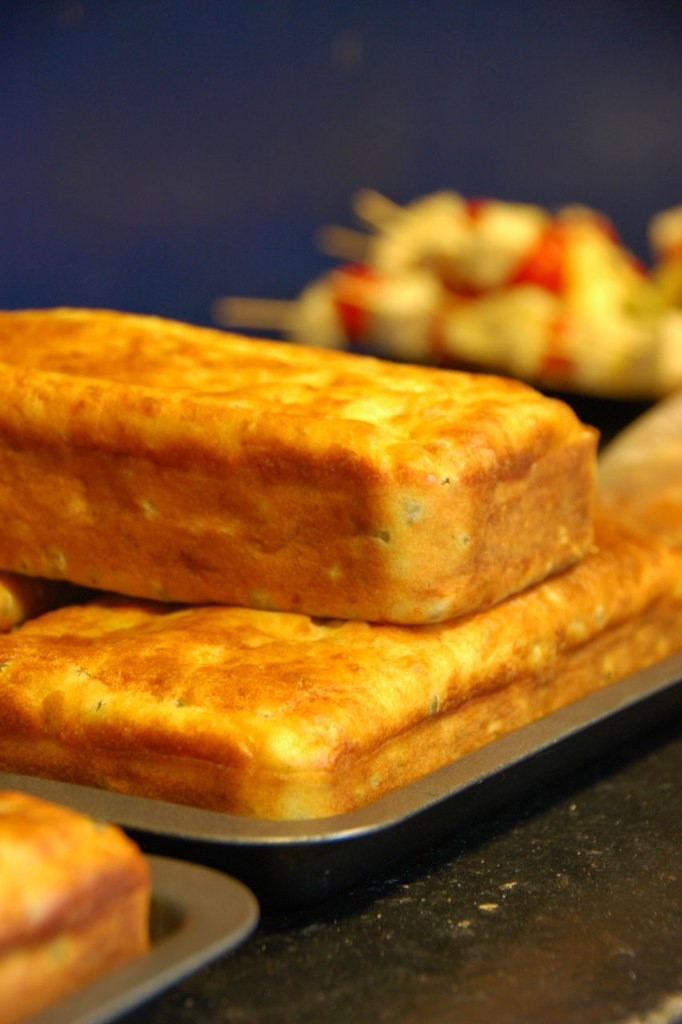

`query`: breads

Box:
[0,305,682,1024]
